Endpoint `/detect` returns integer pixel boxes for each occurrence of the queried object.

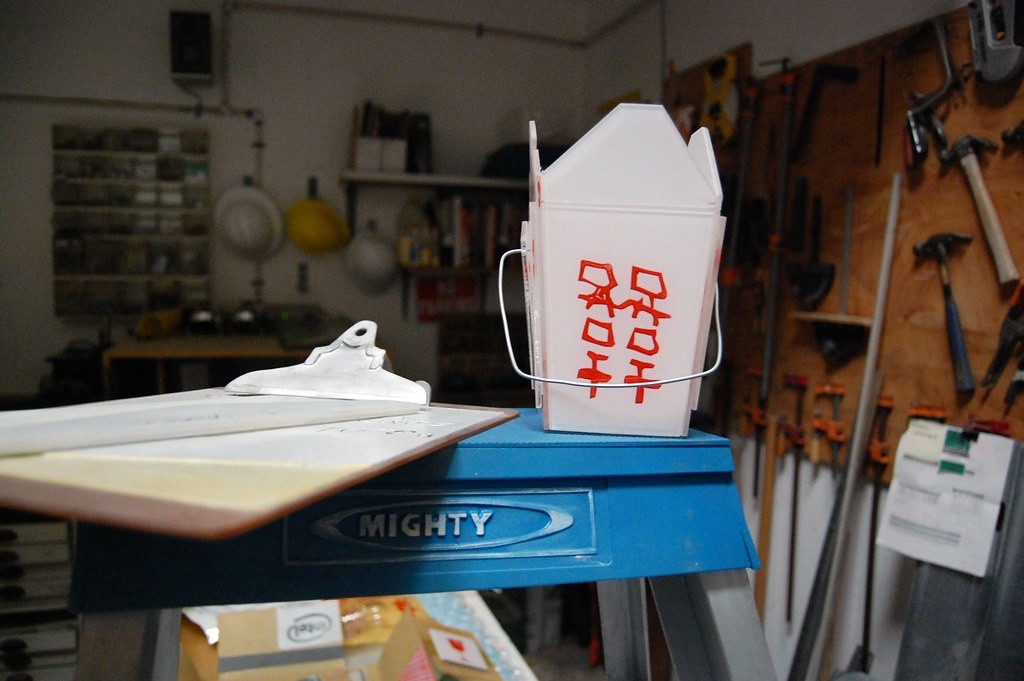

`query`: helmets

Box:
[213,184,284,259]
[285,199,350,252]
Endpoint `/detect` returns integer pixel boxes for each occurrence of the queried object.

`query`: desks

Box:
[100,333,396,394]
[66,408,775,680]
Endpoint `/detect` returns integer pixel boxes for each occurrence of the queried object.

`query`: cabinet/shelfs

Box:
[51,124,214,320]
[341,169,527,321]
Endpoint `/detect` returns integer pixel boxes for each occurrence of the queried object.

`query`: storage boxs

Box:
[376,612,503,681]
[177,597,429,681]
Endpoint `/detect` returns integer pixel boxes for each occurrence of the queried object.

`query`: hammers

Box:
[910,231,977,400]
[937,135,1020,287]
[1001,120,1023,144]
